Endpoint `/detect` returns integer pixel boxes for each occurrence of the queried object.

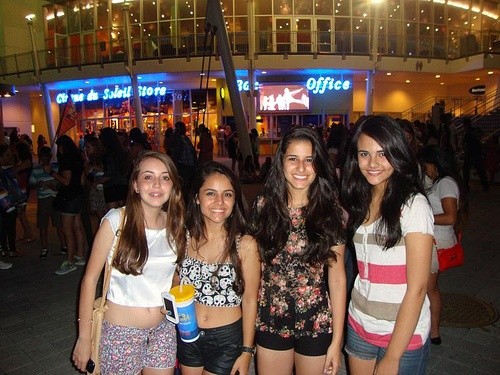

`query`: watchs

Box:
[242,345,257,356]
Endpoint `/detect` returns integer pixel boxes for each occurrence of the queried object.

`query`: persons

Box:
[72,150,186,375]
[160,162,261,375]
[344,113,434,375]
[249,125,349,375]
[416,144,459,346]
[0,118,261,275]
[288,100,489,194]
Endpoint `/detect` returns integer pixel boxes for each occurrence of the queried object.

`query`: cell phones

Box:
[86,358,95,374]
[161,291,179,324]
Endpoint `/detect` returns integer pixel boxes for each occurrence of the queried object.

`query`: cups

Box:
[169,284,200,343]
[95,171,104,190]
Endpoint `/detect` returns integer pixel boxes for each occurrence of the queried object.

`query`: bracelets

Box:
[77,318,93,323]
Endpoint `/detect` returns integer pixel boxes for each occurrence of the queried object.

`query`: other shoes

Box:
[60,245,67,254]
[430,335,442,345]
[1,248,9,257]
[9,248,20,257]
[72,255,86,265]
[39,247,49,260]
[0,261,14,269]
[54,260,77,275]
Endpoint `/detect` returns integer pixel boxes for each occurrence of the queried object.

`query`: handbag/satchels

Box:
[85,305,106,375]
[436,242,465,272]
